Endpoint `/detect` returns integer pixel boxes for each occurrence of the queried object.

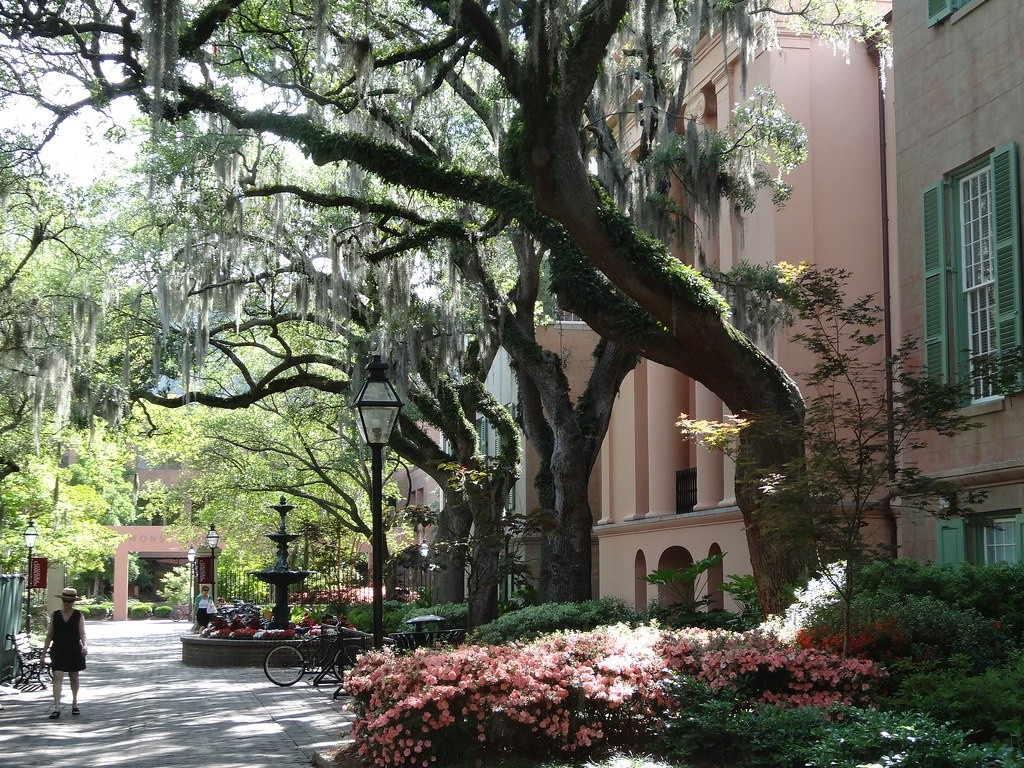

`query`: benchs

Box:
[6,630,54,690]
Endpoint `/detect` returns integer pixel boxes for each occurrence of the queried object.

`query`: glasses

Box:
[62,599,75,604]
[203,590,208,591]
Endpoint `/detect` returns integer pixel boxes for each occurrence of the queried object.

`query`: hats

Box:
[54,588,82,600]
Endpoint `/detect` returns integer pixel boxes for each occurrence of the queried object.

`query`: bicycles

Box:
[263,614,365,687]
[170,603,194,622]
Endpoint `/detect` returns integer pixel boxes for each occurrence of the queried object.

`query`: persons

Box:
[41,588,88,719]
[192,586,213,635]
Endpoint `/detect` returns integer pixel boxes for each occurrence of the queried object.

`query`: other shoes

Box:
[49,711,61,719]
[72,708,80,715]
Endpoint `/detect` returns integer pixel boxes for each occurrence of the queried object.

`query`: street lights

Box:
[23,518,38,634]
[356,353,401,654]
[187,544,196,622]
[206,523,220,604]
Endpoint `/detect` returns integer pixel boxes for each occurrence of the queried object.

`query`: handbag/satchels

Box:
[207,594,217,614]
